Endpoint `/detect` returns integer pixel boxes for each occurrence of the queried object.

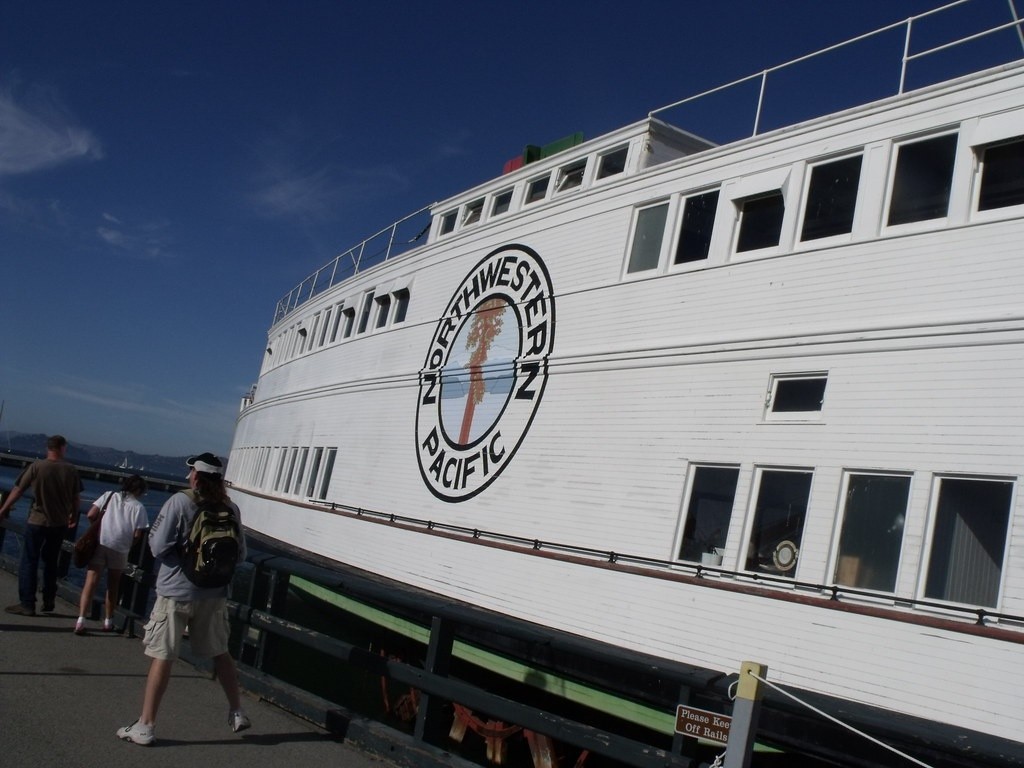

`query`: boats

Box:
[119,457,127,469]
[215,0,1024,768]
[114,462,119,467]
[139,467,144,471]
[127,465,134,469]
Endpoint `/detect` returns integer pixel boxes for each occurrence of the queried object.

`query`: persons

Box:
[73,474,149,634]
[0,435,84,615]
[116,453,252,745]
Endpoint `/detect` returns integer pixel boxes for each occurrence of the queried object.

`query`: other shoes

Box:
[104,623,113,631]
[4,604,37,616]
[41,604,53,611]
[74,623,86,633]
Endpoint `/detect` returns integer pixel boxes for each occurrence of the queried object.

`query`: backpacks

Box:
[180,488,241,588]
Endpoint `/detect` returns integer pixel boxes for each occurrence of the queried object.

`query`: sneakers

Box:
[116,716,156,745]
[228,708,250,732]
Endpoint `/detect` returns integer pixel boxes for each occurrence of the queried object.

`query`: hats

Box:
[186,452,224,476]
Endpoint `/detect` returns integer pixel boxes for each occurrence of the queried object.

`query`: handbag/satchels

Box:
[73,491,115,569]
